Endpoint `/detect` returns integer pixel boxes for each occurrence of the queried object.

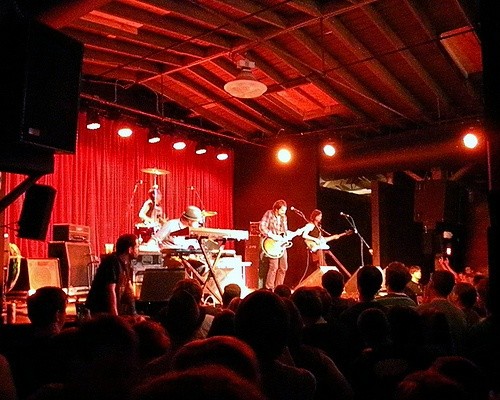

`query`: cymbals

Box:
[202,210,217,216]
[140,168,170,174]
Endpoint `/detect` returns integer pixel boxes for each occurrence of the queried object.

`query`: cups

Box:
[104,243,114,254]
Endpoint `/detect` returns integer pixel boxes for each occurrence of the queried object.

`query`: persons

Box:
[147,206,210,290]
[85,234,145,322]
[0,258,500,400]
[139,188,166,244]
[259,200,301,291]
[303,209,339,269]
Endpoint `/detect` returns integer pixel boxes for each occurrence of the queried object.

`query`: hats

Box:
[183,206,202,222]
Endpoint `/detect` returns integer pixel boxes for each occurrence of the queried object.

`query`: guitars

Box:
[304,229,353,252]
[262,224,315,258]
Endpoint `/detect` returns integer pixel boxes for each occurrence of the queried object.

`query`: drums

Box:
[134,224,154,243]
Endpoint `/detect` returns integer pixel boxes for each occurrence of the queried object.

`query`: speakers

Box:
[0,18,85,154]
[18,184,57,240]
[294,265,388,293]
[413,180,471,228]
[7,242,94,292]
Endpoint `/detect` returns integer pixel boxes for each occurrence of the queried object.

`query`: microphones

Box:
[291,206,301,213]
[340,212,351,217]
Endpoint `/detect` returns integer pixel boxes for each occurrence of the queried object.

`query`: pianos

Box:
[160,225,249,304]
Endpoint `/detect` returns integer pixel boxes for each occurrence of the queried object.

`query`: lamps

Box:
[78,100,490,165]
[224,51,268,98]
